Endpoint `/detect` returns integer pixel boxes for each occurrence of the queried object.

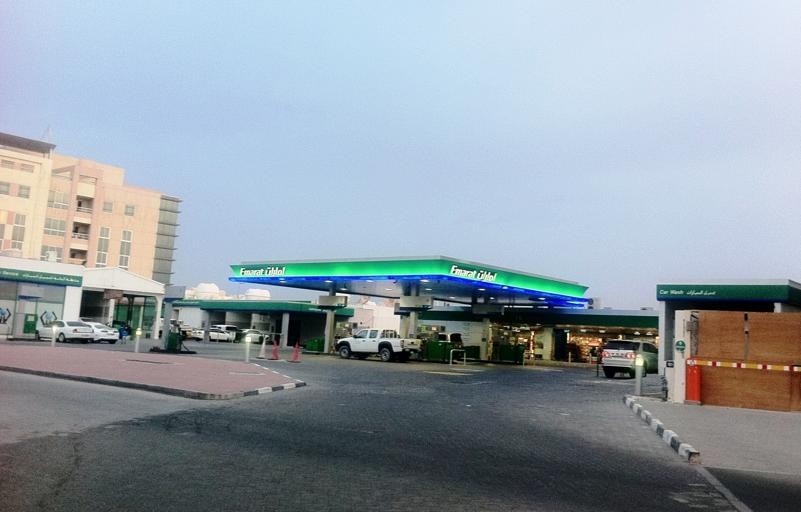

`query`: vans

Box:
[415,331,463,362]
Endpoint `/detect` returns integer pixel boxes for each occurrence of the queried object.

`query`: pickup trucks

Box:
[335,328,422,362]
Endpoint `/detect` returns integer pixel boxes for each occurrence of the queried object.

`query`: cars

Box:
[191,324,271,344]
[601,339,658,378]
[34,320,120,344]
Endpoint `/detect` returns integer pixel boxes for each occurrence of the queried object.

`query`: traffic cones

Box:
[268,339,281,360]
[256,338,267,359]
[286,340,301,363]
